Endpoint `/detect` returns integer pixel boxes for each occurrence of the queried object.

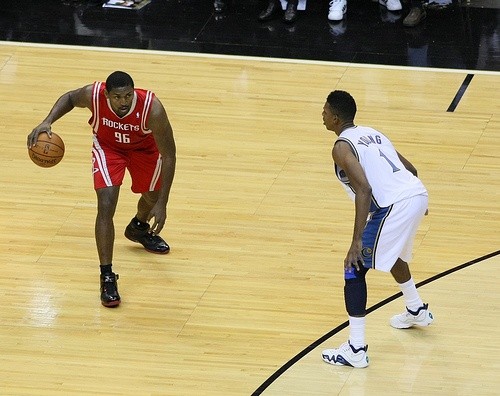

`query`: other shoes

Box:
[403,5,427,28]
[214,0,226,13]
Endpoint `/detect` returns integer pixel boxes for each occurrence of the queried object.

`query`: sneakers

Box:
[328,0,348,21]
[322,340,370,368]
[379,0,402,11]
[124,217,170,254]
[390,303,433,329]
[100,269,121,307]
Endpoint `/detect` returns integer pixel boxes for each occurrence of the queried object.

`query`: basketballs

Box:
[28,132,65,168]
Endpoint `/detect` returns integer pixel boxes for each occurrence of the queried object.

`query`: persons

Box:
[319,89,437,369]
[27,69,176,307]
[211,0,428,24]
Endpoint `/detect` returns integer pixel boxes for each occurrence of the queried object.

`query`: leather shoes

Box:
[282,2,298,25]
[258,2,280,21]
